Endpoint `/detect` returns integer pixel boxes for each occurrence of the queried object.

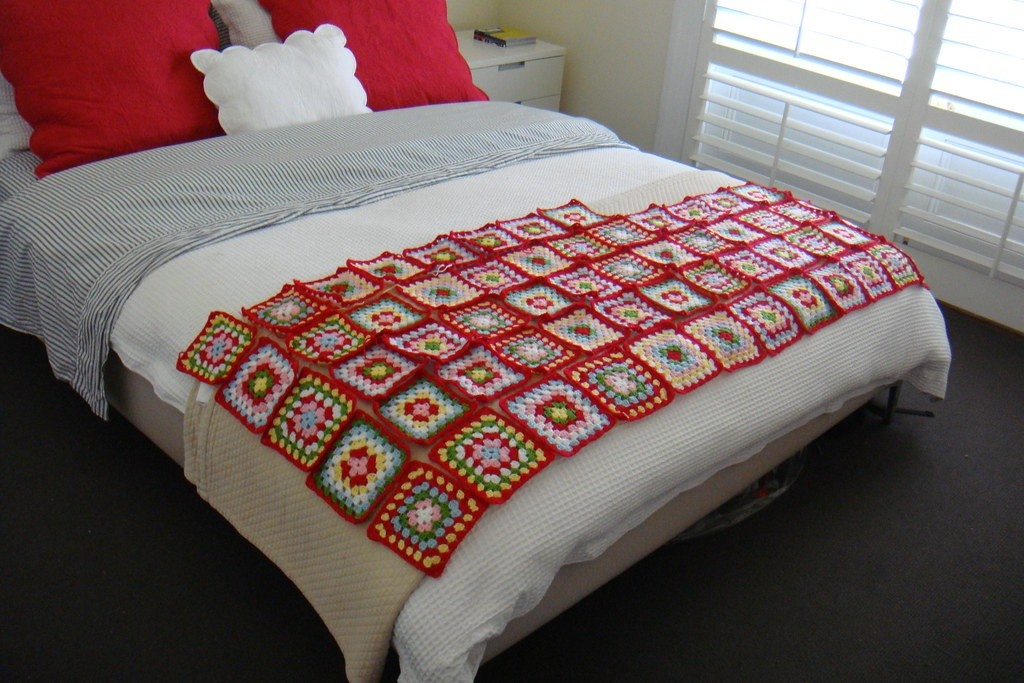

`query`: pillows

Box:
[0,0,489,180]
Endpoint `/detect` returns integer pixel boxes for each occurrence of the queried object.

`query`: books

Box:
[474,27,537,48]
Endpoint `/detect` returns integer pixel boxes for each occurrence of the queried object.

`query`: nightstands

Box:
[453,26,567,113]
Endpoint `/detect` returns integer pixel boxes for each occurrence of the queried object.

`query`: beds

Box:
[0,0,952,683]
